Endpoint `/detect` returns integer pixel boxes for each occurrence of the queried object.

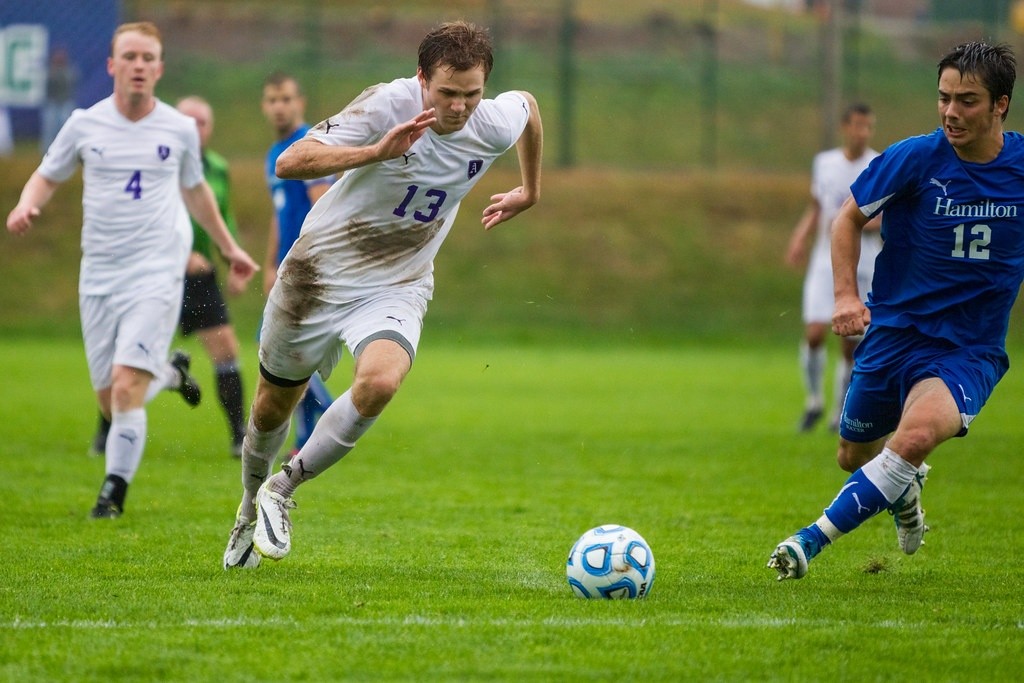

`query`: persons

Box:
[158,92,260,462]
[217,20,543,575]
[785,101,883,435]
[764,37,1023,587]
[253,71,336,467]
[5,21,264,517]
[39,43,79,154]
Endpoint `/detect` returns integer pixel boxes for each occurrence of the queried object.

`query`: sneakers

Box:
[169,346,200,408]
[222,501,263,571]
[91,501,121,519]
[798,403,823,427]
[889,460,933,556]
[766,531,819,581]
[252,477,294,561]
[84,430,108,456]
[231,429,247,459]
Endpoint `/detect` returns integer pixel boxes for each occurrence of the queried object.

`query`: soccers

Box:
[565,522,656,600]
